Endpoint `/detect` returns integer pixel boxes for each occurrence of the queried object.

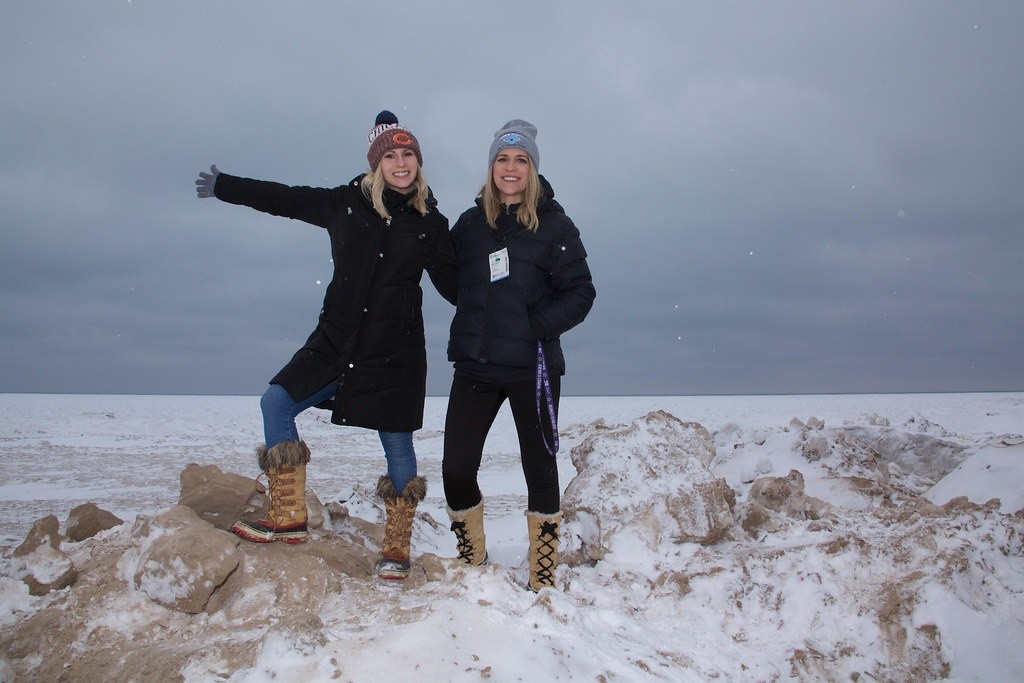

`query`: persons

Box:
[194,110,469,580]
[440,120,597,593]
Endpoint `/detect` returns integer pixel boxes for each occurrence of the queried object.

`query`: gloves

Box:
[196,163,221,198]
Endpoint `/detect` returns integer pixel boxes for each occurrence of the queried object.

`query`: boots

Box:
[232,439,310,543]
[445,496,489,567]
[377,475,429,579]
[523,510,564,593]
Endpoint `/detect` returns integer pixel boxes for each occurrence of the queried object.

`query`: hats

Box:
[489,120,540,171]
[367,110,423,172]
